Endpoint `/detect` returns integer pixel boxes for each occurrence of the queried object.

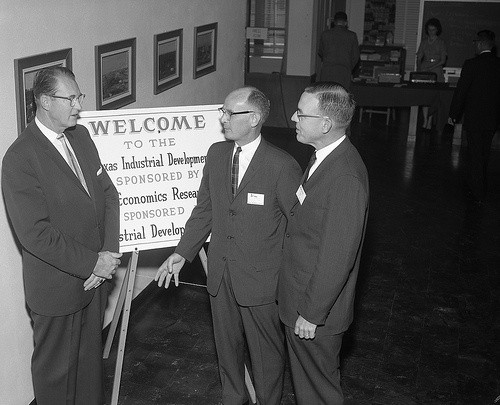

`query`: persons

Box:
[318,11,360,137]
[155,87,303,405]
[448,30,500,210]
[0,67,123,405]
[416,18,448,131]
[276,81,368,405]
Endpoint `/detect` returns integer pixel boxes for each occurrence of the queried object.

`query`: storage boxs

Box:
[409,72,437,84]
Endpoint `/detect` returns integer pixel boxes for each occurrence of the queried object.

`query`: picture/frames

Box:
[14,47,73,138]
[95,36,136,111]
[193,21,218,81]
[153,27,183,95]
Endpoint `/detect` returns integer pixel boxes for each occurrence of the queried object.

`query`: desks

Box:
[347,77,454,138]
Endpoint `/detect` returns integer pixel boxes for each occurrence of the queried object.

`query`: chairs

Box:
[359,66,403,126]
[448,76,459,83]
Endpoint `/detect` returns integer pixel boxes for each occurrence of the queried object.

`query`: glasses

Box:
[218,107,255,117]
[44,93,85,106]
[297,109,321,121]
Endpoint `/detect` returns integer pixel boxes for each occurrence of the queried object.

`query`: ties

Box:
[56,133,91,197]
[303,151,316,185]
[232,146,242,199]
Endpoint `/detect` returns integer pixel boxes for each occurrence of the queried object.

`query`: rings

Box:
[99,279,103,283]
